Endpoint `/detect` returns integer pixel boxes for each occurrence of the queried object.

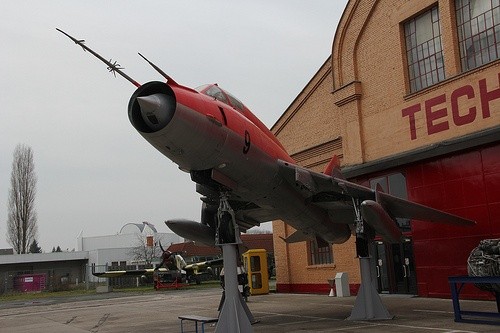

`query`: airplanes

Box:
[91,241,222,285]
[56,28,477,253]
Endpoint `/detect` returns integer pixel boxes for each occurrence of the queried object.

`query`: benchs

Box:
[178,315,218,333]
